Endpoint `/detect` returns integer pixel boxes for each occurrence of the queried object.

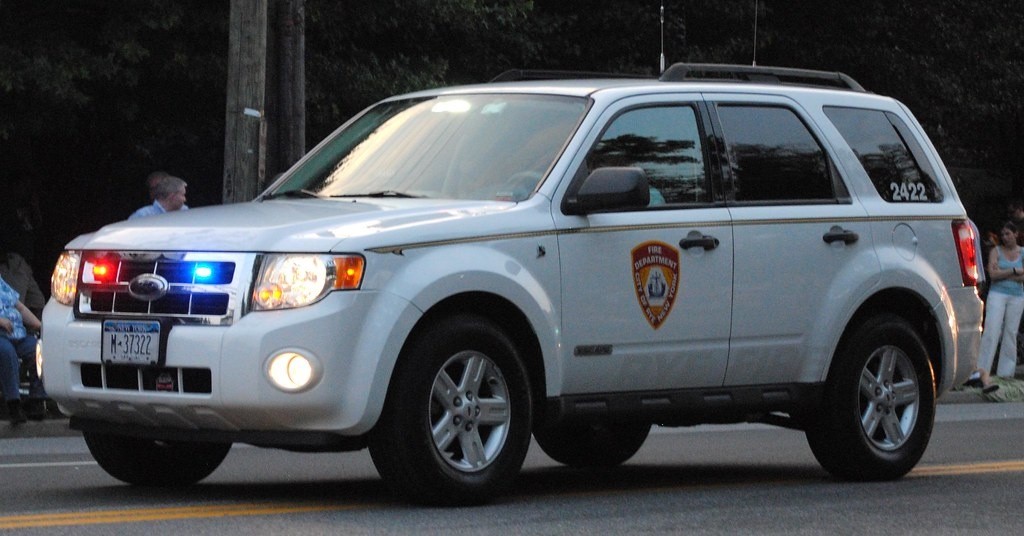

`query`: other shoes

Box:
[28,397,44,421]
[981,383,999,394]
[7,399,27,424]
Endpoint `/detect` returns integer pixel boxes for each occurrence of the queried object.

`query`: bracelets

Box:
[1012,266,1016,274]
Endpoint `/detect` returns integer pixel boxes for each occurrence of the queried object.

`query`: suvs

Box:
[35,61,985,508]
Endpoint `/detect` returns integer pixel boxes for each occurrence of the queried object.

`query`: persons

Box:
[962,201,1024,395]
[566,136,666,204]
[126,173,189,220]
[0,242,61,424]
